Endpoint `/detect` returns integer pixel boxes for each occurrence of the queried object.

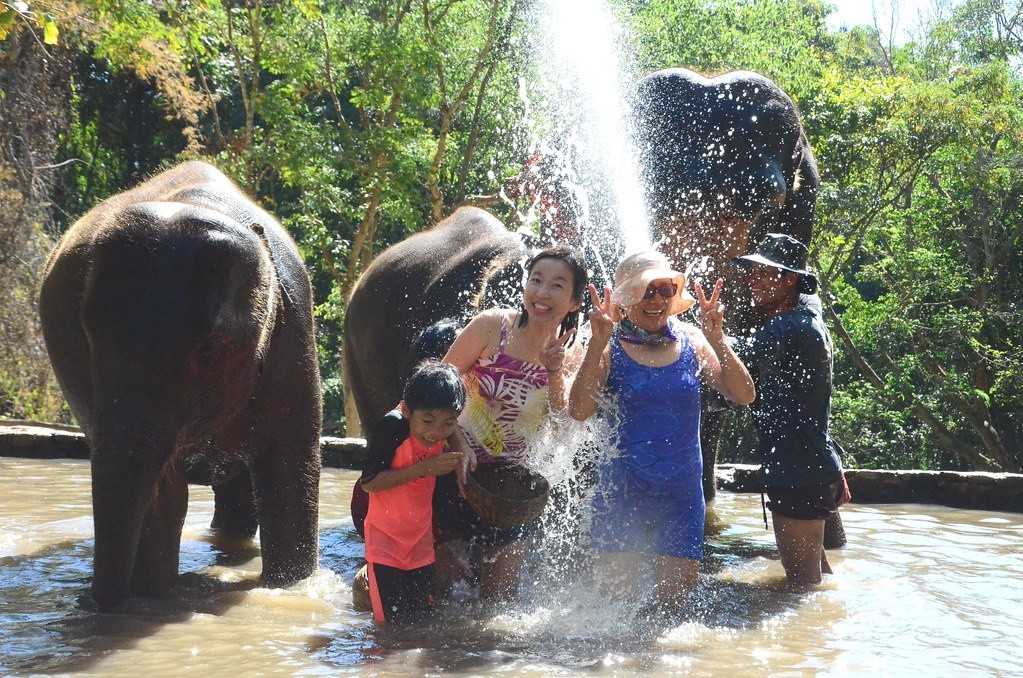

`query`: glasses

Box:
[643,282,678,299]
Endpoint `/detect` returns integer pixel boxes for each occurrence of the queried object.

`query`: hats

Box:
[602,250,696,322]
[734,232,818,295]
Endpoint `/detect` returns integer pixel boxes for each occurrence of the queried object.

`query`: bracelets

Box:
[546,362,563,371]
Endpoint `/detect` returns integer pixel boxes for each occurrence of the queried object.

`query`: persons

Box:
[436,246,588,598]
[731,233,851,583]
[358,360,463,621]
[571,251,756,590]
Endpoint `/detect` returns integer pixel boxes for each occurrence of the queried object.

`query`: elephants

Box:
[341,71,847,619]
[37,159,324,608]
